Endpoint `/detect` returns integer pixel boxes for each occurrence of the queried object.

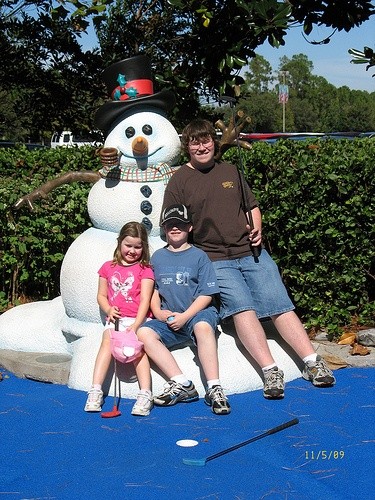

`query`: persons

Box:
[136,204,233,416]
[162,119,337,399]
[82,222,156,417]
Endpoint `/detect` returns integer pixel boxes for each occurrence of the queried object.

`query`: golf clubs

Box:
[101,317,121,418]
[182,418,299,467]
[219,95,260,256]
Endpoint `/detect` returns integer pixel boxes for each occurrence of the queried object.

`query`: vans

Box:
[50,130,104,149]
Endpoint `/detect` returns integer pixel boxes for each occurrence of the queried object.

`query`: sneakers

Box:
[262,366,285,399]
[130,391,154,416]
[204,385,231,414]
[84,388,105,411]
[302,355,337,386]
[153,380,199,406]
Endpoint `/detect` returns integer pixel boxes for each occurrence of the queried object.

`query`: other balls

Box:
[168,316,174,321]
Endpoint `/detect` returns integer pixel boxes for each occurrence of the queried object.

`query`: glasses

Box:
[188,137,213,149]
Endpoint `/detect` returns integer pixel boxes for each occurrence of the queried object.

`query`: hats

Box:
[159,203,190,226]
